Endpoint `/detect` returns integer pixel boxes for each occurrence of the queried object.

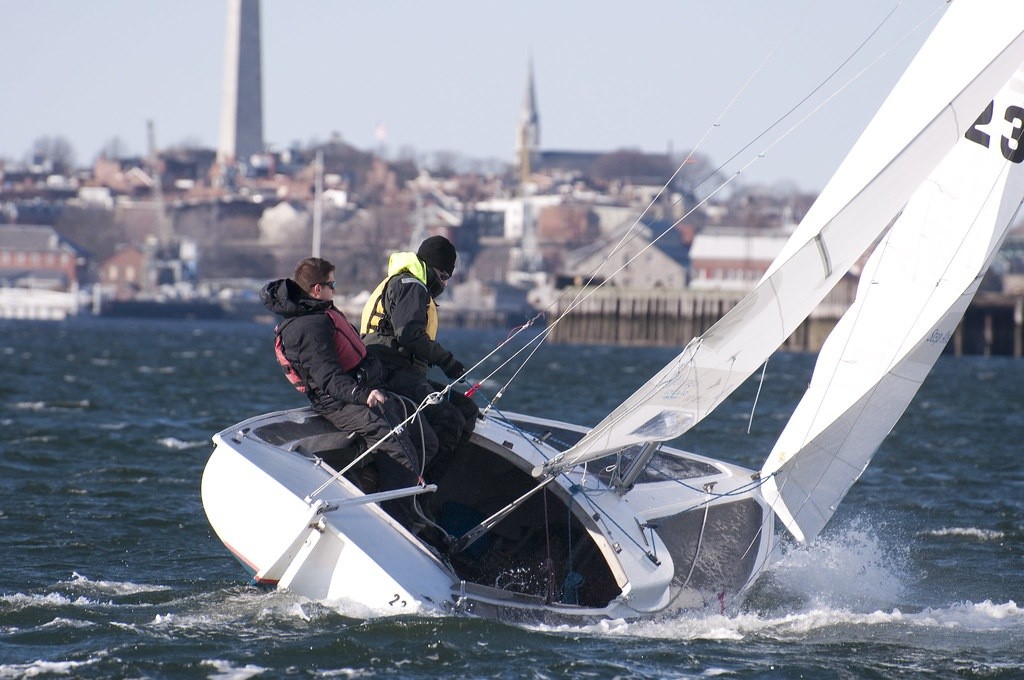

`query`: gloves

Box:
[439,352,467,385]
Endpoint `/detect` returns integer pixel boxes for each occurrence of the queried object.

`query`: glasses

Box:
[310,280,336,289]
[437,268,451,281]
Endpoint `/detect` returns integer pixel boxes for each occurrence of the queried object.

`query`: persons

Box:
[360,234,479,506]
[259,257,440,533]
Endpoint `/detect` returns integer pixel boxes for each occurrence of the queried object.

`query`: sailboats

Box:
[202,2,1024,638]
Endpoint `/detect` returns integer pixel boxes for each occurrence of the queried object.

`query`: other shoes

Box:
[347,459,380,496]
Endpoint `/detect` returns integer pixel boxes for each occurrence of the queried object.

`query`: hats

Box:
[417,234,457,277]
